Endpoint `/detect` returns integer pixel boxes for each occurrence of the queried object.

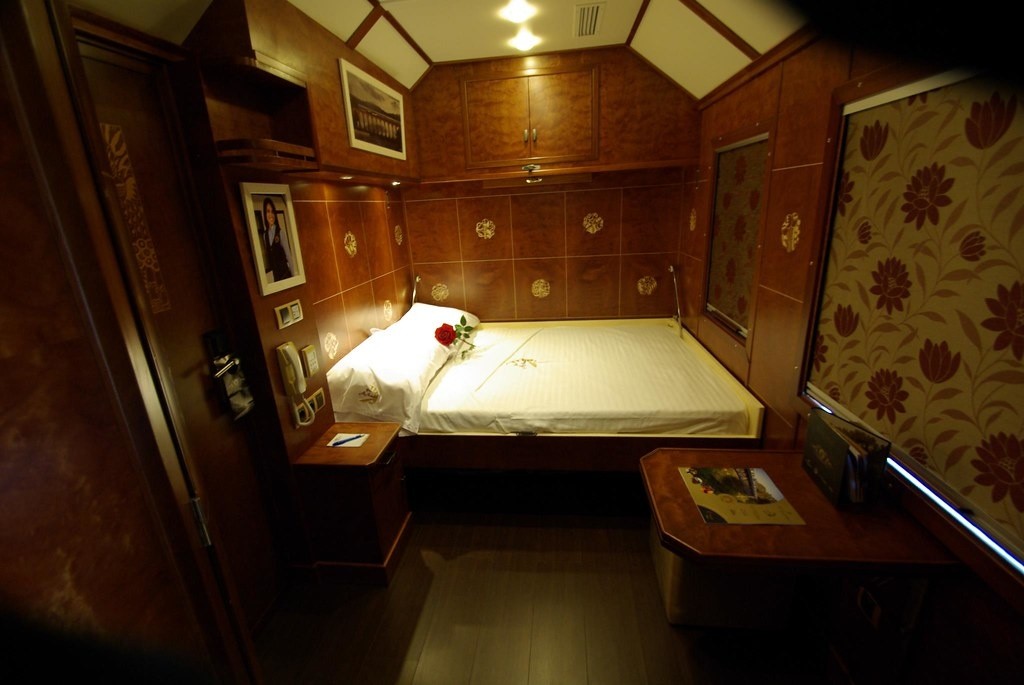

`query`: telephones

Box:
[276,340,308,398]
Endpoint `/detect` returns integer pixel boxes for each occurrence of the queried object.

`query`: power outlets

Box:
[301,343,320,379]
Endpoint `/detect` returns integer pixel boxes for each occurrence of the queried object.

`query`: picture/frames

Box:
[338,58,406,161]
[242,181,307,296]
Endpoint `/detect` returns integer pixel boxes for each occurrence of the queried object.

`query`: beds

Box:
[326,300,762,441]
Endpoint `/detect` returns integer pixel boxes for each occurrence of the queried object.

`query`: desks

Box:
[639,447,958,626]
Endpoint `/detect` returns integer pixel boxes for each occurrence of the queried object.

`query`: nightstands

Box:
[292,420,414,570]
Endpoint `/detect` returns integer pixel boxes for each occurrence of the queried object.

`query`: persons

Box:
[257,196,296,283]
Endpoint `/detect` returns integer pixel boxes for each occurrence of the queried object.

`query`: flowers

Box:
[432,314,478,360]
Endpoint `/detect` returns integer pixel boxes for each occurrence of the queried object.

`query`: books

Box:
[800,406,893,512]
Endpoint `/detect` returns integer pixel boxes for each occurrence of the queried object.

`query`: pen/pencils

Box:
[332,433,366,445]
